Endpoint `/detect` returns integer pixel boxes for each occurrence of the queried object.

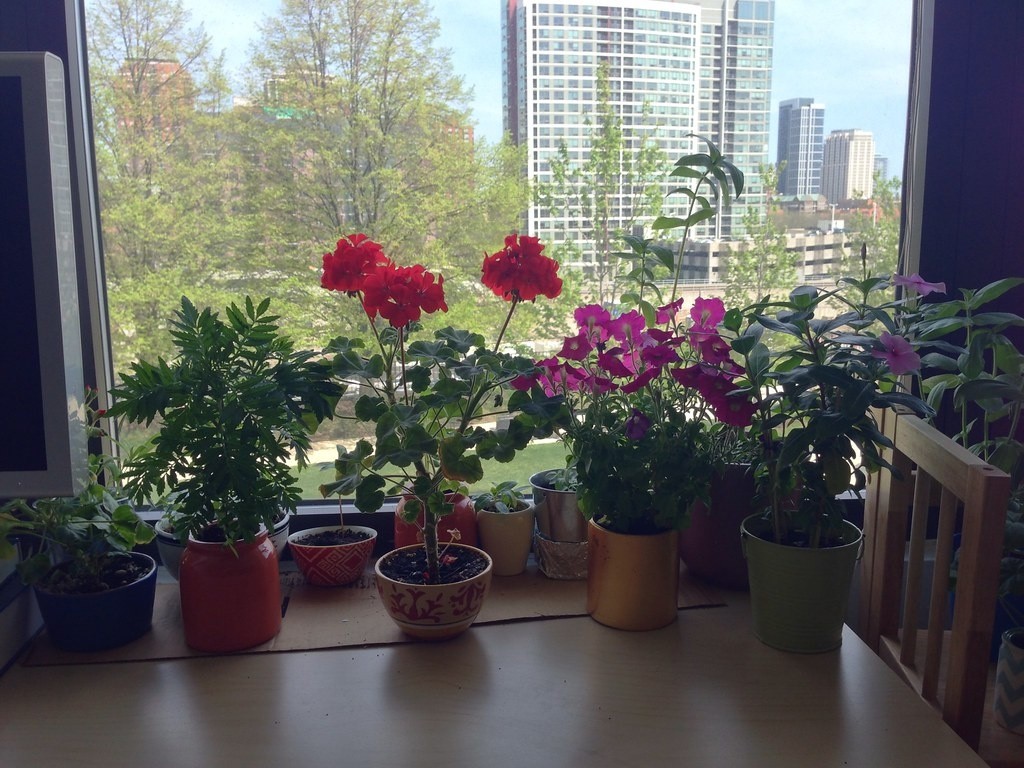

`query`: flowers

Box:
[324,142,948,578]
[84,384,124,498]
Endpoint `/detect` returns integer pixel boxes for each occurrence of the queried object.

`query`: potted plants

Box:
[911,277,1023,735]
[466,480,534,577]
[531,468,589,542]
[0,295,378,653]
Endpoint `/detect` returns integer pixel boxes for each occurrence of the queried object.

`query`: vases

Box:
[678,432,803,587]
[393,491,477,551]
[374,543,494,641]
[32,486,135,514]
[588,513,680,632]
[740,510,864,655]
